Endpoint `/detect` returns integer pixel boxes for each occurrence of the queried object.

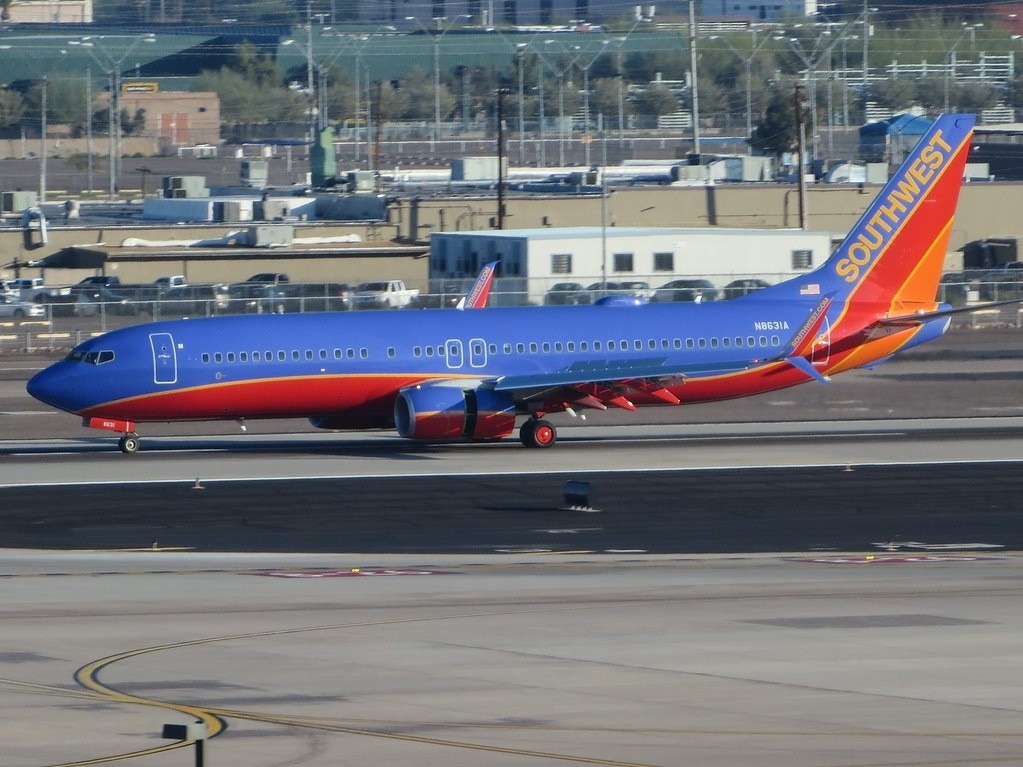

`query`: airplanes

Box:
[24,111,1023,451]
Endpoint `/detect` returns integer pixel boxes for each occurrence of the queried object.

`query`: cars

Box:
[0,273,472,321]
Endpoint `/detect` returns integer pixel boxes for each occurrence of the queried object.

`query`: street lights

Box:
[80,32,157,190]
[0,44,78,204]
[403,14,474,143]
[321,23,400,162]
[67,38,157,194]
[709,30,787,155]
[515,39,611,170]
[546,36,628,167]
